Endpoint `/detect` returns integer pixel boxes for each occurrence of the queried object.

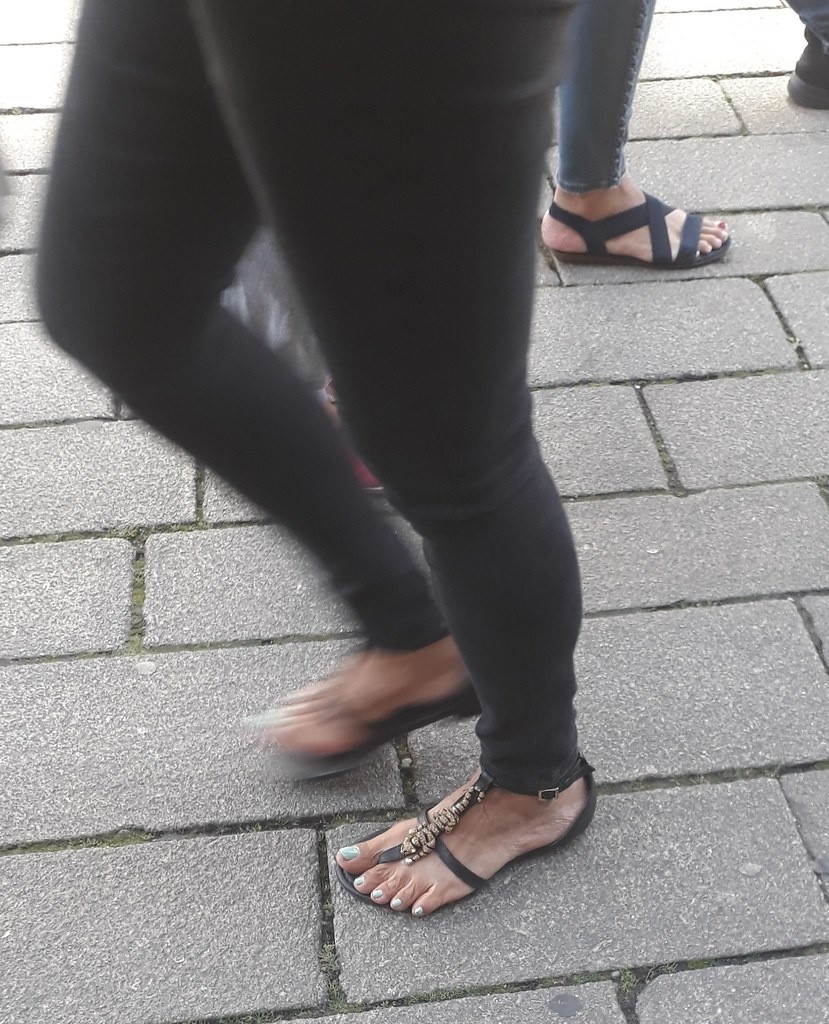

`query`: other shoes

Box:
[787,41,829,110]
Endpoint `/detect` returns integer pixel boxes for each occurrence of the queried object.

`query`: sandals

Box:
[548,188,731,270]
[273,642,484,775]
[335,749,597,908]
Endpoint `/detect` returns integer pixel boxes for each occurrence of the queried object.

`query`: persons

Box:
[35,0,730,917]
[786,0,829,109]
